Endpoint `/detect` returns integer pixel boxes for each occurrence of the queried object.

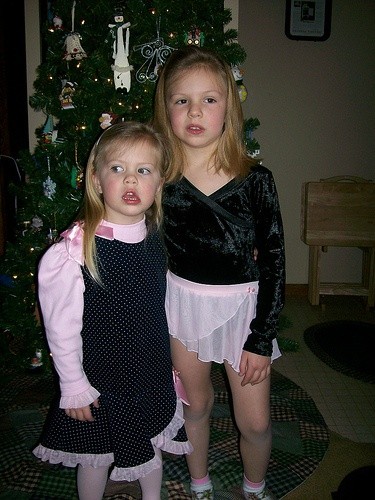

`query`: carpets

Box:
[304,319,375,382]
[0,350,331,500]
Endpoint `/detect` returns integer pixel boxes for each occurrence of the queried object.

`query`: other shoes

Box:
[191,487,214,500]
[242,486,274,500]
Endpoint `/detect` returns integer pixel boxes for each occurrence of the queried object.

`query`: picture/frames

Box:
[284,0,334,43]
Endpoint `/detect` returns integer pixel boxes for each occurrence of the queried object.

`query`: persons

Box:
[155,46,287,500]
[30,123,192,500]
